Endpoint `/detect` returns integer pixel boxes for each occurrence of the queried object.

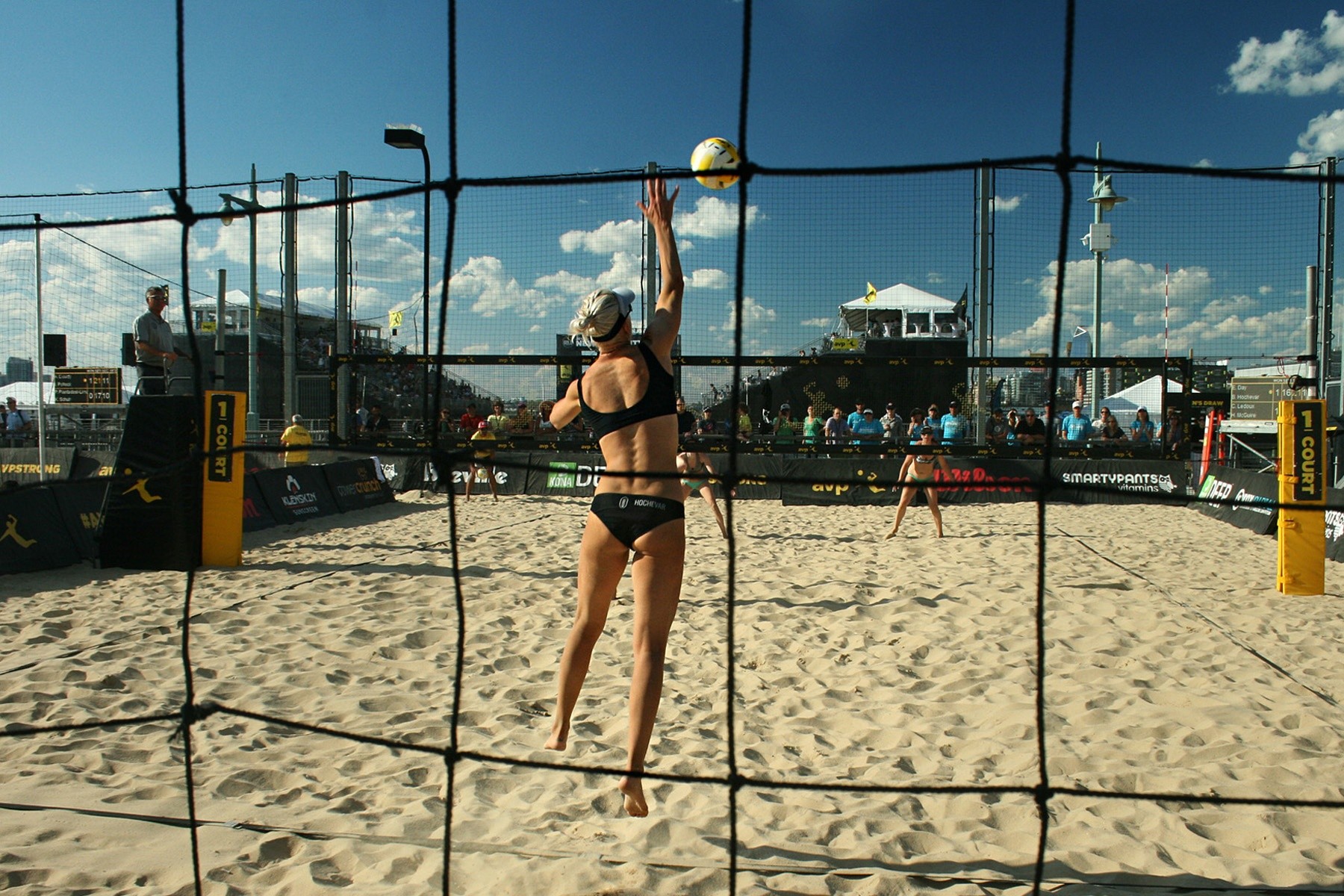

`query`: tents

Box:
[1080,374,1201,438]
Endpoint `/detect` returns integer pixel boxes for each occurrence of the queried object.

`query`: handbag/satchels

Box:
[18,409,35,439]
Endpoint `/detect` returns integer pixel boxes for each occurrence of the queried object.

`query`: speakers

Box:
[122,333,137,366]
[43,334,67,366]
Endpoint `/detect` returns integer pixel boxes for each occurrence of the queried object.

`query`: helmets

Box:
[540,401,556,412]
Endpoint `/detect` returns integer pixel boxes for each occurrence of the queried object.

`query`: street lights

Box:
[1085,141,1130,418]
[380,127,432,441]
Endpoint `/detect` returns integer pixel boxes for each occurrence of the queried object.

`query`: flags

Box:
[952,286,968,322]
[865,281,876,305]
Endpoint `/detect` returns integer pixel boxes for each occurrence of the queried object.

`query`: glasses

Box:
[705,409,712,412]
[442,411,450,414]
[625,303,633,320]
[677,404,684,406]
[518,406,526,409]
[147,295,165,301]
[1101,411,1205,423]
[7,402,12,404]
[1027,414,1034,417]
[921,431,932,435]
[887,405,894,410]
[494,404,501,407]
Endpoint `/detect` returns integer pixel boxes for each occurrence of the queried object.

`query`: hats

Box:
[1044,400,1051,406]
[703,406,712,413]
[1136,406,1147,413]
[592,287,636,342]
[1072,401,1082,408]
[466,404,476,408]
[910,401,958,434]
[780,404,791,411]
[855,398,873,414]
[993,408,1003,414]
[6,398,15,403]
[478,422,488,429]
[292,414,303,424]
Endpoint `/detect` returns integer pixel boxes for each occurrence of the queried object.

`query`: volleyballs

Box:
[690,137,743,190]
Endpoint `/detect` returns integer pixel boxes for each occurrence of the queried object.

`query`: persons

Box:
[1129,405,1154,448]
[676,332,839,443]
[907,401,966,446]
[891,425,956,538]
[0,396,32,448]
[464,421,498,502]
[543,177,684,818]
[439,398,586,441]
[984,400,1128,448]
[676,437,728,539]
[278,414,312,468]
[824,406,851,445]
[772,403,798,445]
[1156,406,1205,451]
[801,404,826,458]
[846,398,884,445]
[879,402,905,445]
[133,286,177,395]
[355,396,393,431]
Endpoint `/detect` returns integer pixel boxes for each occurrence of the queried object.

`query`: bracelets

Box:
[543,428,545,431]
